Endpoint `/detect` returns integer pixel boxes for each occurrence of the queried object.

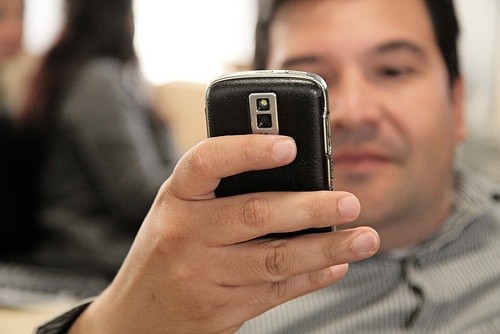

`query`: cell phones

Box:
[206,70,334,240]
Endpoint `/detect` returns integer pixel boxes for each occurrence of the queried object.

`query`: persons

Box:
[17,0,500,334]
